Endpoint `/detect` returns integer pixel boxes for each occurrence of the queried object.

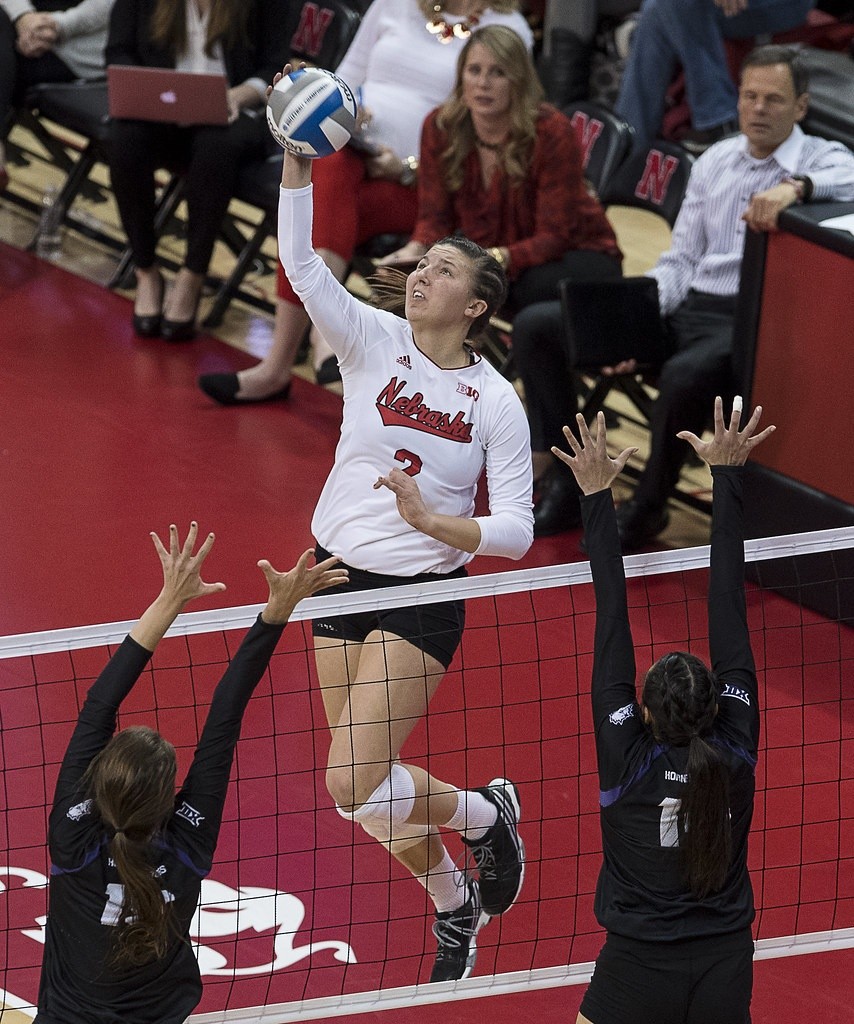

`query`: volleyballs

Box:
[266,67,358,160]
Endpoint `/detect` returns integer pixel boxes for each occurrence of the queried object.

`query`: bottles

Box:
[37,188,62,261]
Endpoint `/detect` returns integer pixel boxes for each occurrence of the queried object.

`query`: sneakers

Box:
[461,777,525,915]
[428,877,493,984]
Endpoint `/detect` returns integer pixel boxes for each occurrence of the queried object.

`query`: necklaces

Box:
[476,133,499,152]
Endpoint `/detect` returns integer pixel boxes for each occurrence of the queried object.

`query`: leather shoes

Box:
[580,499,670,553]
[534,489,581,538]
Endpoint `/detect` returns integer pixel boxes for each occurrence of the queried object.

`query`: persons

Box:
[551,394,777,1024]
[264,61,527,984]
[29,521,349,1024]
[532,43,853,554]
[0,0,816,453]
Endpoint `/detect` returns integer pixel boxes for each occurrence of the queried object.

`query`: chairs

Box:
[0,0,719,566]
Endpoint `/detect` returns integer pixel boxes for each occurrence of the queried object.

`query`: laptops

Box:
[107,65,230,126]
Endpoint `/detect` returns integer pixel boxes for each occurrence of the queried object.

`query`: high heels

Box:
[133,271,164,337]
[161,284,199,340]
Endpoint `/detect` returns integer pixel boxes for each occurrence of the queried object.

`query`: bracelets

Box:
[491,247,508,273]
[782,174,813,205]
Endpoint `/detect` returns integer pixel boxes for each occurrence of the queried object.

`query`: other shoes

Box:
[199,366,291,406]
[316,354,342,384]
